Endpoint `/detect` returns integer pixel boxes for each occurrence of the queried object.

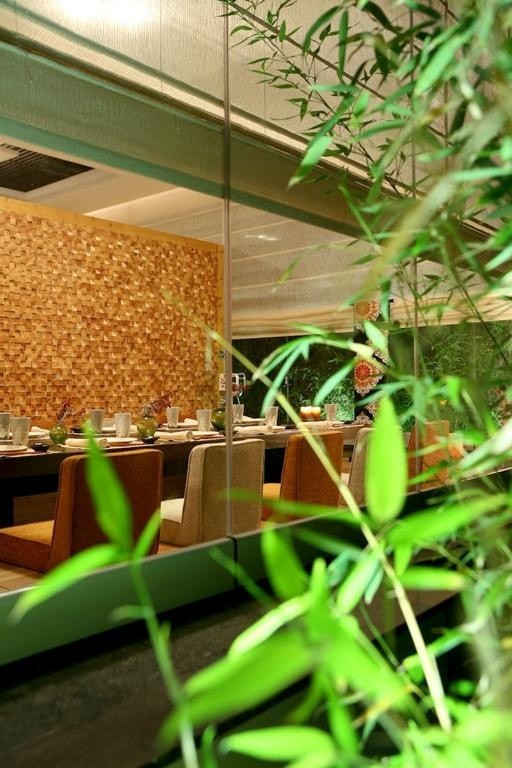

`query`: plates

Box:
[4,418,286,454]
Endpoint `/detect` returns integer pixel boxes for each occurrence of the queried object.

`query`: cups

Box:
[324,405,336,421]
[1,410,10,440]
[9,416,31,446]
[165,405,179,428]
[113,412,132,438]
[265,406,278,426]
[89,408,105,434]
[194,409,212,431]
